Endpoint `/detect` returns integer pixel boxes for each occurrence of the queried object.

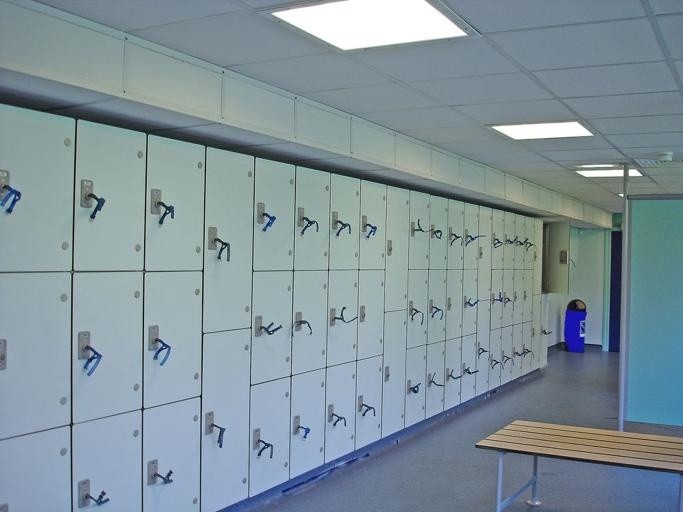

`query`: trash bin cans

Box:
[564,299,587,353]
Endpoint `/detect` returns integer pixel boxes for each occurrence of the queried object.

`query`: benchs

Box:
[475,419,683,512]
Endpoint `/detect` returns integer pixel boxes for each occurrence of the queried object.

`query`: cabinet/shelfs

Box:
[295,141,553,481]
[143,273,202,404]
[201,328,250,512]
[0,272,71,442]
[141,396,201,512]
[250,273,292,386]
[147,133,204,273]
[0,102,75,273]
[254,158,295,272]
[0,424,73,512]
[70,408,143,511]
[76,118,146,271]
[201,147,251,332]
[72,270,144,427]
